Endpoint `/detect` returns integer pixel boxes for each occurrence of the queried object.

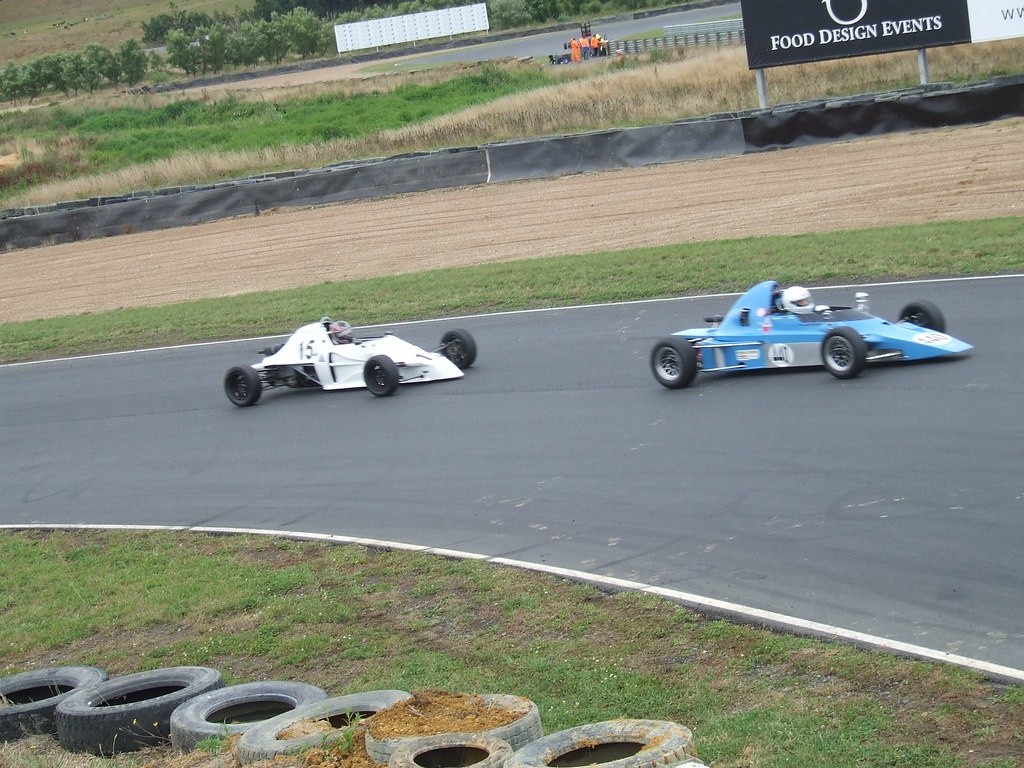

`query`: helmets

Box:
[782,286,815,314]
[334,321,351,337]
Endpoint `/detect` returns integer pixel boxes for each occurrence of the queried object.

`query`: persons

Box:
[571,36,580,63]
[580,33,589,60]
[591,34,607,57]
[781,286,831,321]
[329,320,362,344]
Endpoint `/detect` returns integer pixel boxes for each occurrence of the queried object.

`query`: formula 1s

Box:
[649,279,975,390]
[223,316,477,407]
